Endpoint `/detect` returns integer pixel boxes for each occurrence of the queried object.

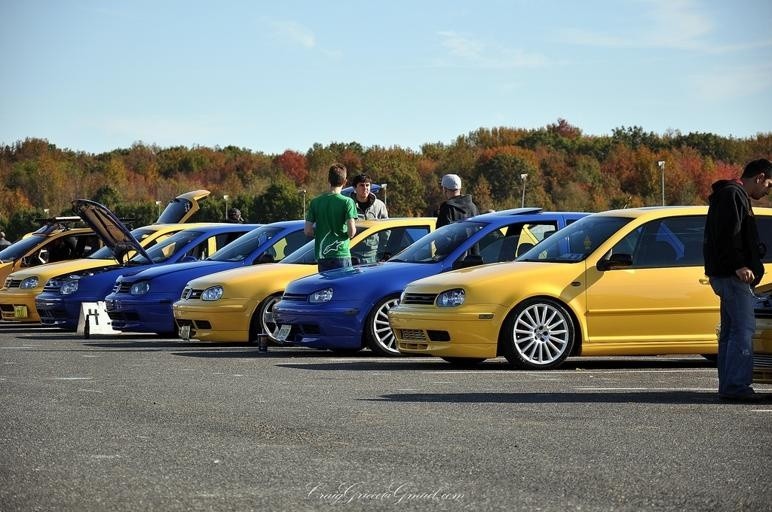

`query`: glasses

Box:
[749,273,771,302]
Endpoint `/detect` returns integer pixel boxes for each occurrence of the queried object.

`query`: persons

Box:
[0,231,13,253]
[222,208,249,223]
[701,156,771,403]
[304,162,360,273]
[432,172,479,258]
[344,172,393,267]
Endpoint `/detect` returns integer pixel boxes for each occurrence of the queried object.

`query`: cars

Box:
[0,189,772,385]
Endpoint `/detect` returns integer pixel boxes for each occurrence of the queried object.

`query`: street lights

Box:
[381,184,389,205]
[656,160,667,206]
[520,173,528,208]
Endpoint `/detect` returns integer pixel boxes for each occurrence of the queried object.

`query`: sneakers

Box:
[719,389,760,402]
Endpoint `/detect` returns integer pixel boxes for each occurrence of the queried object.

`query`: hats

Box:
[440,174,461,189]
